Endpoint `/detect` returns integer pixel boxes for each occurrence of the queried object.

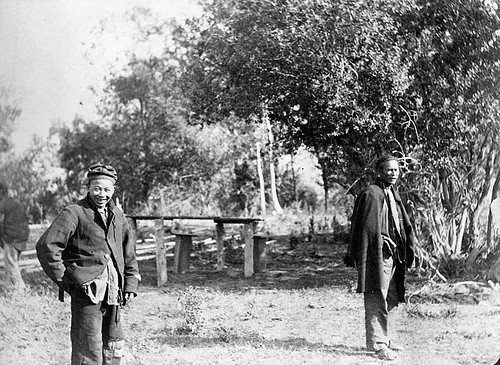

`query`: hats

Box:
[87,163,119,184]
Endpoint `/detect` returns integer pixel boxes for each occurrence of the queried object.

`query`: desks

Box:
[124,215,264,288]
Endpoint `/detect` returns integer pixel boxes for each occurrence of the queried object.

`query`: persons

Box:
[344,155,415,360]
[36,165,141,365]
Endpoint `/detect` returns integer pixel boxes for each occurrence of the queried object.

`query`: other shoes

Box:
[366,348,397,360]
[390,342,402,351]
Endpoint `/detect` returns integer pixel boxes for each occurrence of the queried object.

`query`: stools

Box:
[171,232,198,275]
[253,234,275,274]
